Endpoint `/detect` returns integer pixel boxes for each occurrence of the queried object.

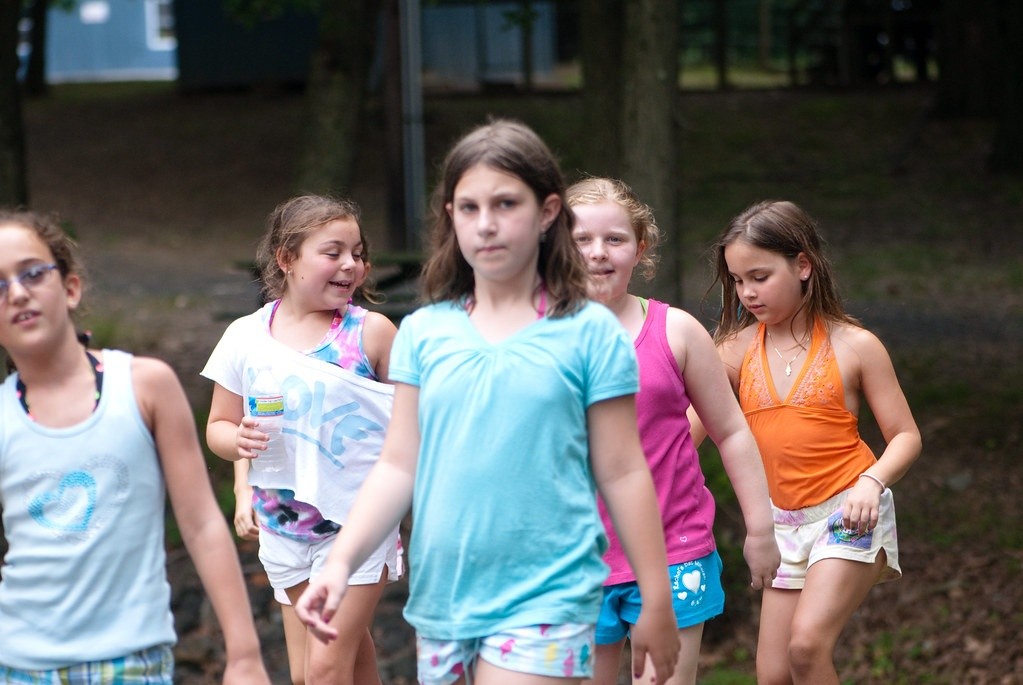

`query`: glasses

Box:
[0,263,65,306]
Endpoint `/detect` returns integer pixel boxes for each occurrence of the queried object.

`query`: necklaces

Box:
[766,328,811,376]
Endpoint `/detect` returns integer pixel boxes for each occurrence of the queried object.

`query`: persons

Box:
[566,179,782,685]
[206,194,402,685]
[294,122,682,685]
[686,201,922,685]
[0,207,273,685]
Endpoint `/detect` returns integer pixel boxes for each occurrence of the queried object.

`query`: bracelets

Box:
[859,473,885,494]
